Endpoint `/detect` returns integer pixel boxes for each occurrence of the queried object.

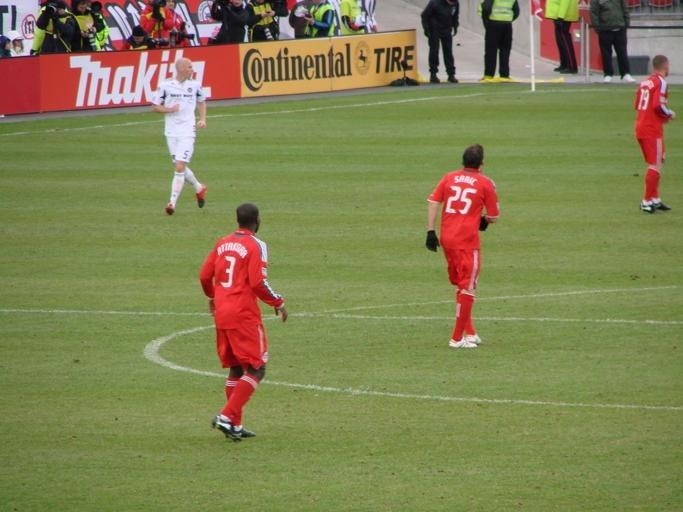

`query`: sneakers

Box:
[429,71,441,83]
[164,202,176,216]
[553,64,578,74]
[448,332,483,348]
[194,184,207,210]
[603,74,613,83]
[621,72,637,84]
[639,200,673,213]
[447,73,458,82]
[210,414,257,441]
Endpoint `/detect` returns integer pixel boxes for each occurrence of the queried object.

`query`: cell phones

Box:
[267,12,270,13]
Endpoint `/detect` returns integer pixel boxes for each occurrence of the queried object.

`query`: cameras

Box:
[178,21,195,40]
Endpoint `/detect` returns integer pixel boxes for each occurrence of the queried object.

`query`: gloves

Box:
[424,229,442,253]
[479,215,488,231]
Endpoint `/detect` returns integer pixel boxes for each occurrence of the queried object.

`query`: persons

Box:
[210,1,378,46]
[199,203,288,443]
[151,57,207,215]
[0,29,30,58]
[30,1,193,55]
[633,55,676,213]
[421,0,460,83]
[477,0,520,82]
[544,0,579,74]
[589,0,636,83]
[425,143,501,349]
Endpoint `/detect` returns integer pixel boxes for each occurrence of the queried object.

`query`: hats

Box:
[132,26,145,37]
